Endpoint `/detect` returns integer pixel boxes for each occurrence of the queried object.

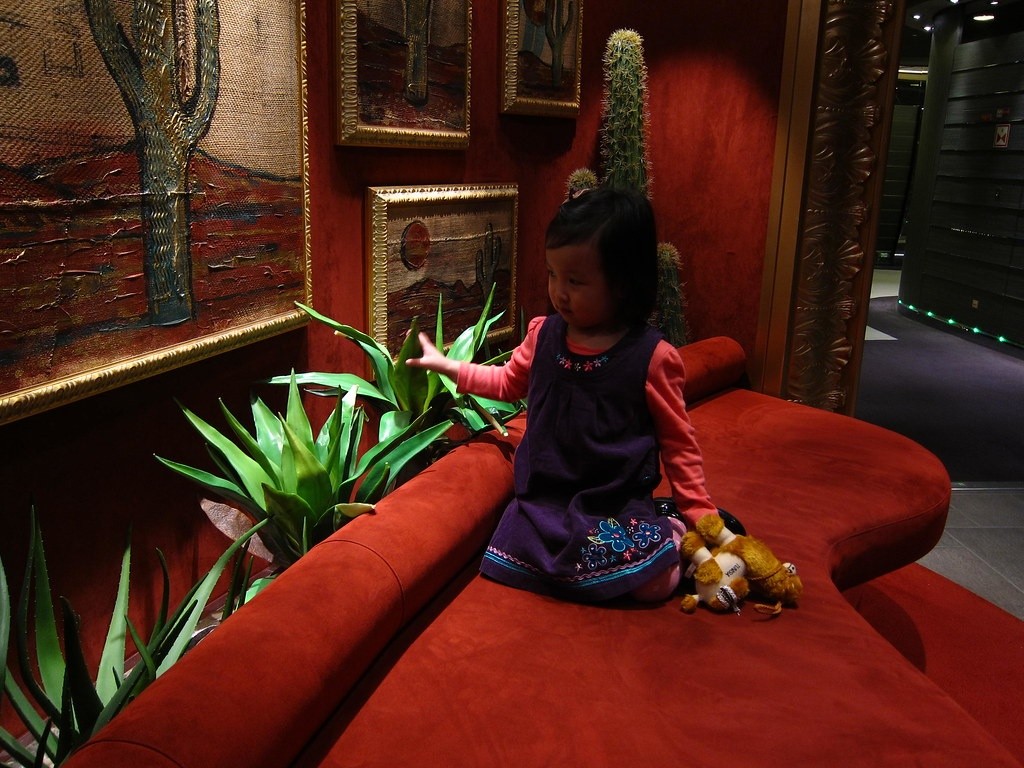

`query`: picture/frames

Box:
[499,0,587,125]
[331,0,474,153]
[0,0,319,428]
[356,176,527,383]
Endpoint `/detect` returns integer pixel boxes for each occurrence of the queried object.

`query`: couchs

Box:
[56,333,1024,768]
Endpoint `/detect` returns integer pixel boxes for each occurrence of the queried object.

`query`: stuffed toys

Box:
[681,514,803,615]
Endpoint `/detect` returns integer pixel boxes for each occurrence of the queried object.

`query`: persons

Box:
[405,188,746,603]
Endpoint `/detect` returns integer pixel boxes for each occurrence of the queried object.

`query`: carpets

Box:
[834,559,1024,768]
